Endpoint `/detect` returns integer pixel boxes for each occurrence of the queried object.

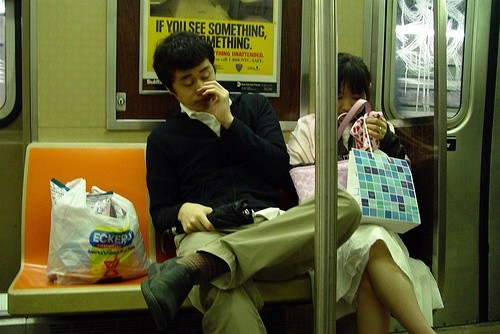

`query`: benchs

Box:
[8,141,312,317]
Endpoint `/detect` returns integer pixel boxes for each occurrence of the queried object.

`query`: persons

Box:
[287,52,443,334]
[141,31,363,334]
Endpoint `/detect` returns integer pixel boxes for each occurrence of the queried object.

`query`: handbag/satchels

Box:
[346,117,421,234]
[289,160,349,206]
[46,178,152,284]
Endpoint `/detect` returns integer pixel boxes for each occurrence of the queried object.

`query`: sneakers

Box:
[141,256,201,331]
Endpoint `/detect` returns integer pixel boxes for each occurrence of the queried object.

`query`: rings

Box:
[378,126,382,134]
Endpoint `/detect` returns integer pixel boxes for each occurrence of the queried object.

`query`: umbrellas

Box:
[161,199,254,255]
